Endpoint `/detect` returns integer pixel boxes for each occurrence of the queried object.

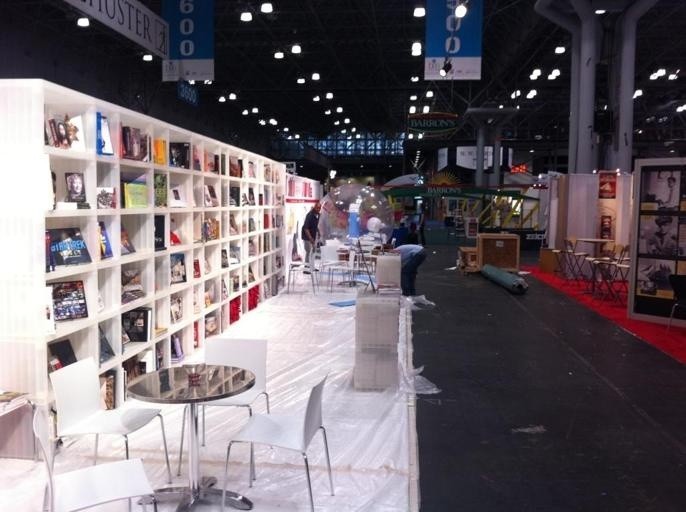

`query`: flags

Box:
[423,0,484,81]
[161,0,215,83]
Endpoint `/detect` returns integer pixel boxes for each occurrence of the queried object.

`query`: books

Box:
[119,122,167,401]
[263,161,284,299]
[96,113,117,410]
[43,109,91,377]
[170,141,199,362]
[228,152,260,326]
[636,166,686,300]
[203,150,227,339]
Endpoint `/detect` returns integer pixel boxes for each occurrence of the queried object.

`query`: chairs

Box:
[32,407,158,512]
[667,274,686,329]
[177,338,270,480]
[551,234,631,306]
[48,356,173,484]
[220,372,335,512]
[287,244,384,297]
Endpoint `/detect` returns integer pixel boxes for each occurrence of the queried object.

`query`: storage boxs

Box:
[476,232,521,273]
[457,247,480,272]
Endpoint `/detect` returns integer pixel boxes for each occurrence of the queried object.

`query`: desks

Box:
[126,365,256,512]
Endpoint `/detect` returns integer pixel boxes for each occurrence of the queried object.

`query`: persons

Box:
[390,244,427,296]
[419,203,427,245]
[300,202,322,274]
